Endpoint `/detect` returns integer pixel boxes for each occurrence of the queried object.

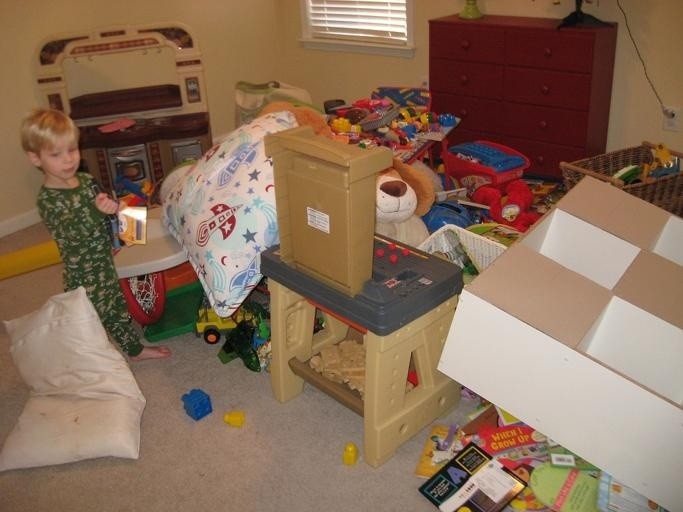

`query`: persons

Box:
[21,108,173,364]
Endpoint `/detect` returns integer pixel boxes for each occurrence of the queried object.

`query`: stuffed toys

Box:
[367,146,436,249]
[256,103,349,145]
[603,140,678,187]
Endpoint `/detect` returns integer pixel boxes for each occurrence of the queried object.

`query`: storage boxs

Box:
[415,223,507,282]
[437,176,682,510]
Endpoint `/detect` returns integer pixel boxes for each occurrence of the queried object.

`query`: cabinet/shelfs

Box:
[427,10,617,182]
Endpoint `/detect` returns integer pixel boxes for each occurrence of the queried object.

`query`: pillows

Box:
[162,110,300,319]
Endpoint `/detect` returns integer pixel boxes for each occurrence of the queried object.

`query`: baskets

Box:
[559,141,683,218]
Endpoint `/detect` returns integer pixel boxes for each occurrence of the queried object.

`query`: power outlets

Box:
[660,106,681,132]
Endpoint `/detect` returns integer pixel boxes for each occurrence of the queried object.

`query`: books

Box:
[415,407,669,511]
